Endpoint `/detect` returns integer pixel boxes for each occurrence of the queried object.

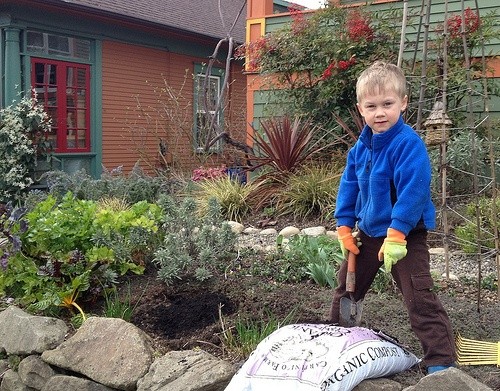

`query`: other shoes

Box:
[428,363,455,375]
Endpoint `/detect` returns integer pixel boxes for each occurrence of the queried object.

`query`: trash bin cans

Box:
[224,166,247,187]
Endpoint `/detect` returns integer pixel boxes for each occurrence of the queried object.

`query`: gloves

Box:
[336,226,362,262]
[378,228,407,273]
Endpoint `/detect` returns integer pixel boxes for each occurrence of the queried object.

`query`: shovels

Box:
[338,236,363,328]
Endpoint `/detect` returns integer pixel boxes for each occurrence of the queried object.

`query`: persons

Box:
[330,58,458,375]
